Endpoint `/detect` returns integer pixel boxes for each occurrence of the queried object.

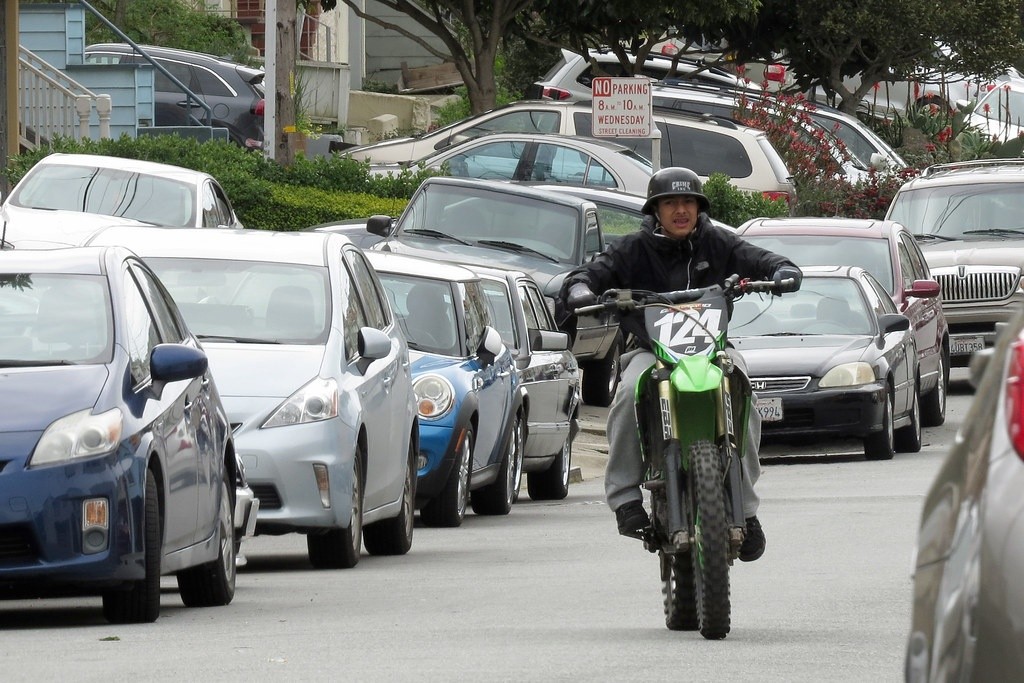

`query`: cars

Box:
[0,243,250,628]
[729,215,952,455]
[1,151,244,270]
[903,298,1024,683]
[284,28,1023,528]
[73,229,422,570]
[725,265,924,462]
[82,40,264,157]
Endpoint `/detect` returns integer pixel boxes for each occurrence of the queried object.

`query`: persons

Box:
[558,166,803,563]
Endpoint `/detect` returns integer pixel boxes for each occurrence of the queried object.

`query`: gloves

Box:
[566,282,600,318]
[770,265,802,297]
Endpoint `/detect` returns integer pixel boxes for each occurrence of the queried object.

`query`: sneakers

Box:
[738,515,766,562]
[615,500,650,535]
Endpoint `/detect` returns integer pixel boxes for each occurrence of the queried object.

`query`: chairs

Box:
[264,285,316,342]
[849,311,871,333]
[727,302,767,336]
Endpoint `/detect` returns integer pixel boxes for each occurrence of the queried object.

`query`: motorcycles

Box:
[566,268,797,642]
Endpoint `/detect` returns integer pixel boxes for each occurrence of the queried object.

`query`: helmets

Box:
[640,167,710,216]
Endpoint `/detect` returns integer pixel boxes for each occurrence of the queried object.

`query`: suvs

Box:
[877,155,1023,362]
